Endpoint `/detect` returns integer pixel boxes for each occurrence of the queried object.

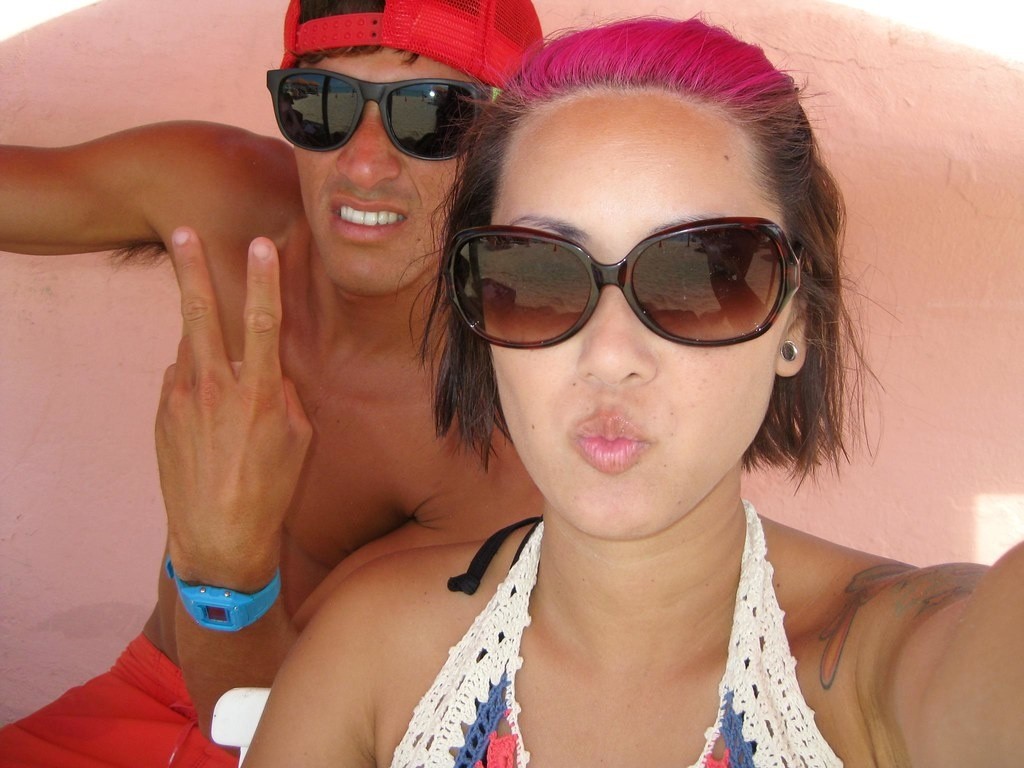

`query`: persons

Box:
[243,19,1024,768]
[0,0,548,768]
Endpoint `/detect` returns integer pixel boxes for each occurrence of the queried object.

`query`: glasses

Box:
[442,216,804,350]
[266,69,493,161]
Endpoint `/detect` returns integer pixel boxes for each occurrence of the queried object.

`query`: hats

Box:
[279,0,543,91]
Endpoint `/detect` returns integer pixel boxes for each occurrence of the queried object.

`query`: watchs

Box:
[165,556,281,632]
